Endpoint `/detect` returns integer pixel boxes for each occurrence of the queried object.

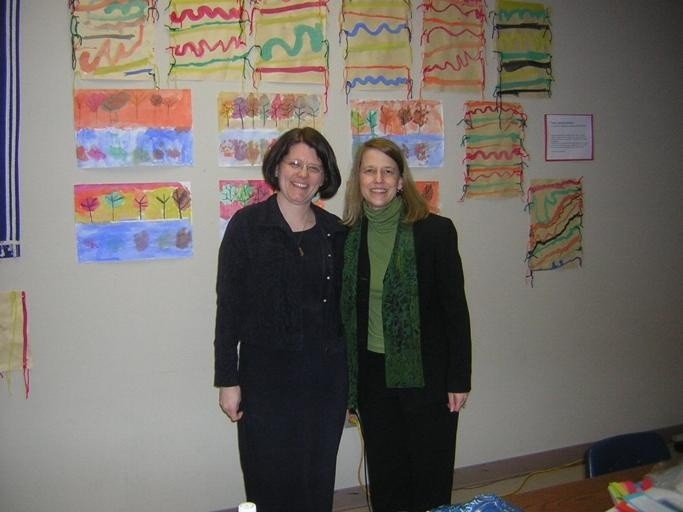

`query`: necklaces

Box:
[279,210,313,258]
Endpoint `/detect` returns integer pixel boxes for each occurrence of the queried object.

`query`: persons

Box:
[209,125,350,511]
[335,135,473,509]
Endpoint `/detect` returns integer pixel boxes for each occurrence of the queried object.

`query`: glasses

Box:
[286,159,321,176]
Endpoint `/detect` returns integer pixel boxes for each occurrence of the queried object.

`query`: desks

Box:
[501,455,683,512]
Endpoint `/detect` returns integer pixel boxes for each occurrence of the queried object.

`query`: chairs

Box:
[584,432,672,478]
